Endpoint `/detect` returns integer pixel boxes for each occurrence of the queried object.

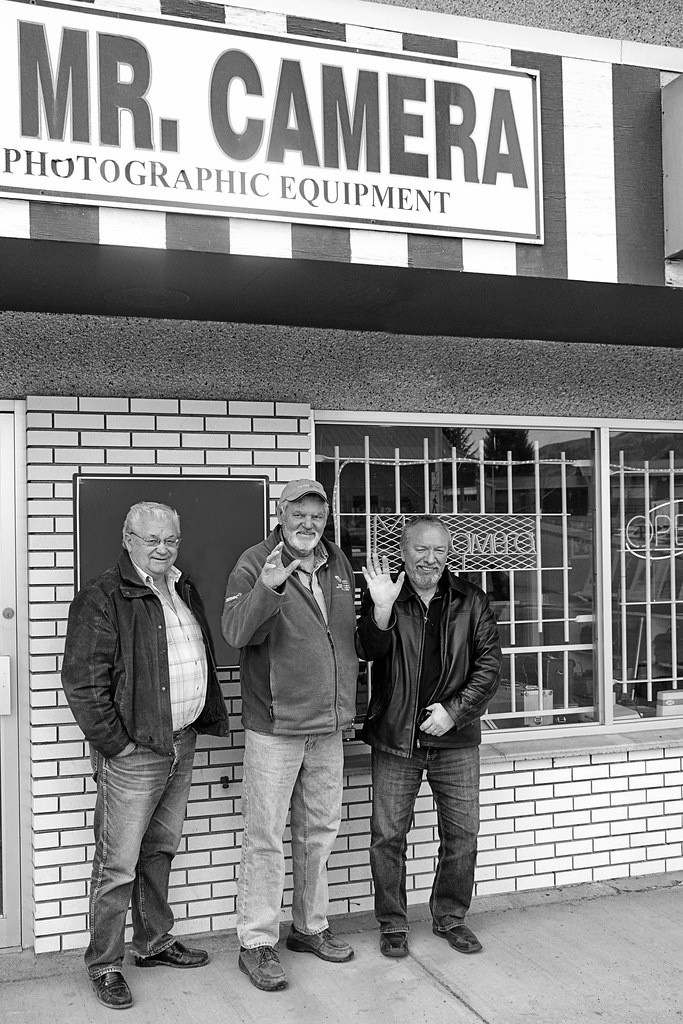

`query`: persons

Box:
[356,515,503,957]
[221,479,354,992]
[61,502,228,1008]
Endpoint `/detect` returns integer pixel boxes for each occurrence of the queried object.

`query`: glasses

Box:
[128,532,182,548]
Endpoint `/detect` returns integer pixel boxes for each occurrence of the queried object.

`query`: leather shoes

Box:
[134,941,209,967]
[92,970,132,1009]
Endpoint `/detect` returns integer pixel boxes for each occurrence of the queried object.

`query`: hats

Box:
[280,479,328,503]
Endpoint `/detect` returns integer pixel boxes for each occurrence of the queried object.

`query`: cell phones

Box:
[417,708,432,725]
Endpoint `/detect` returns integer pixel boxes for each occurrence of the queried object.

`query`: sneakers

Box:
[432,924,483,954]
[238,944,287,991]
[380,931,409,956]
[286,924,354,963]
